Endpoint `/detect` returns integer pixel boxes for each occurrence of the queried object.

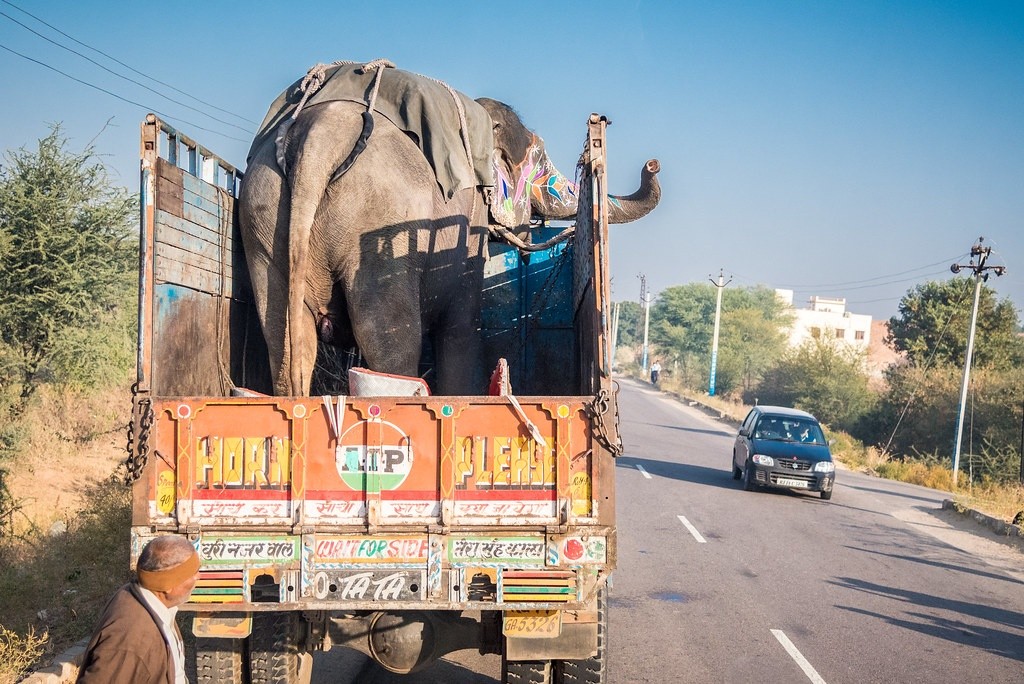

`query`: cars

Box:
[732,405,835,500]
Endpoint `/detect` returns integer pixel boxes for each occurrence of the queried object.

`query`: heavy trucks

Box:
[129,113,625,684]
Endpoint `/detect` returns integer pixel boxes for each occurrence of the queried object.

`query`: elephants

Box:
[236,58,663,397]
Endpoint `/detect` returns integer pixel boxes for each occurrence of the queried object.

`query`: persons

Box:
[76,535,201,684]
[759,419,774,431]
[795,422,816,443]
[772,420,786,436]
[651,360,661,384]
